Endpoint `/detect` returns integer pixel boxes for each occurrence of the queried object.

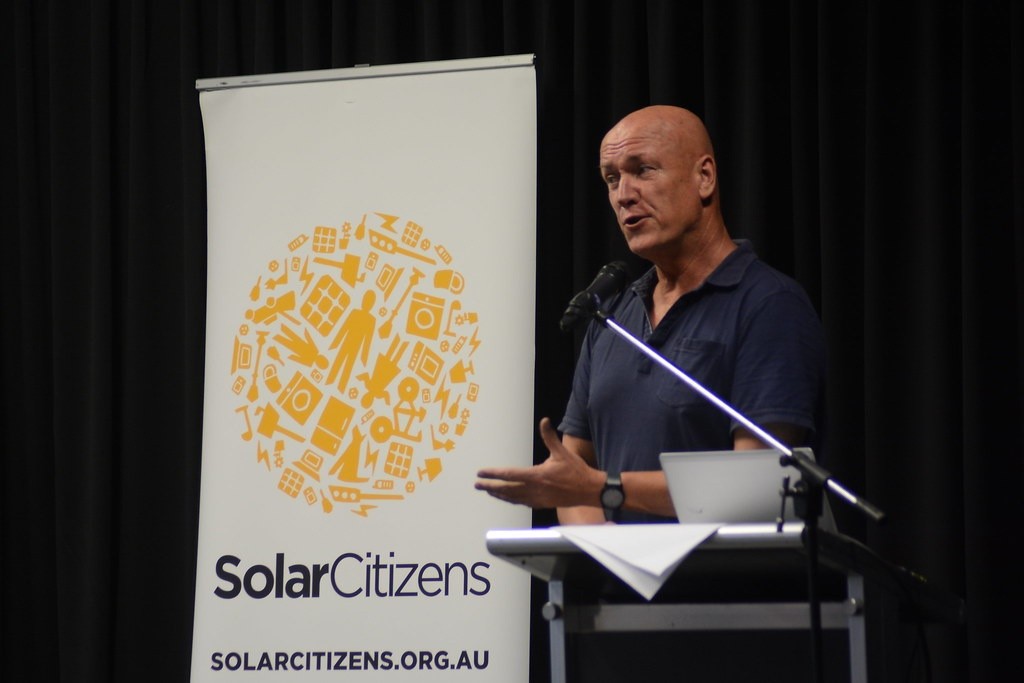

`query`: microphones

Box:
[559,260,628,333]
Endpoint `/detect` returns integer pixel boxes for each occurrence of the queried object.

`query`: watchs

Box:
[600,470,625,511]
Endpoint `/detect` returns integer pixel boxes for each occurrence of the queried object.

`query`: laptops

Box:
[659,448,839,533]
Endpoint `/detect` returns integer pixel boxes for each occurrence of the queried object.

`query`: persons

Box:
[476,104,827,525]
